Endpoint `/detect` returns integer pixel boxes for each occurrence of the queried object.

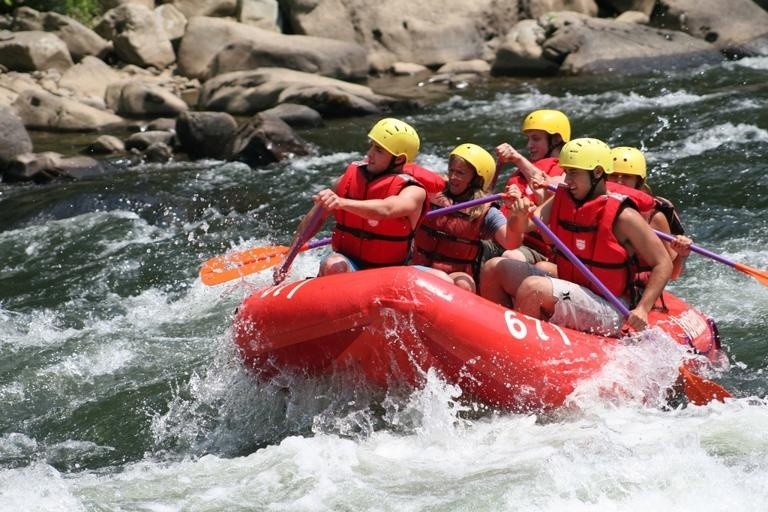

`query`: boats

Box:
[232,266,715,422]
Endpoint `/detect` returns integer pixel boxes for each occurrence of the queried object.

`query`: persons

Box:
[272,118,454,289]
[413,143,522,295]
[535,147,691,280]
[497,137,673,339]
[474,109,571,268]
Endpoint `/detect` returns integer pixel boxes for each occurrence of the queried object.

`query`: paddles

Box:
[538,180,767,304]
[528,210,733,408]
[202,192,510,287]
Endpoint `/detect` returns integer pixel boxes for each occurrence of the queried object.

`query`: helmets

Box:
[448,143,496,191]
[558,138,615,176]
[367,118,420,173]
[522,110,571,158]
[613,147,647,189]
